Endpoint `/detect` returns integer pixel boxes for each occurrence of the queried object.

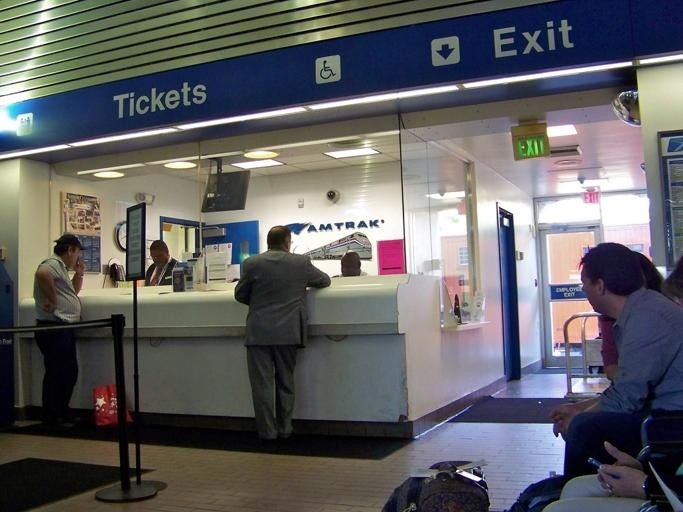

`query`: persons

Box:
[331,250,368,278]
[33,233,86,437]
[144,240,184,286]
[541,241,682,511]
[234,225,331,450]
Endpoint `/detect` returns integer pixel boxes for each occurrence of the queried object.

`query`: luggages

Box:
[380,461,490,512]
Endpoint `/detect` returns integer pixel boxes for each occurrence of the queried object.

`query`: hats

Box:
[54,234,88,250]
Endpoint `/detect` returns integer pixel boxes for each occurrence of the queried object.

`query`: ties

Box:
[150,266,162,287]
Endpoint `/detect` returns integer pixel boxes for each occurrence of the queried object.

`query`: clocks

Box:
[114,220,127,252]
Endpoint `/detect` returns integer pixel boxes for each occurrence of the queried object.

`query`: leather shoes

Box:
[42,417,80,431]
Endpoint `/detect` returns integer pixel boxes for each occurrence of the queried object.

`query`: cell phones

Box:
[587,458,601,467]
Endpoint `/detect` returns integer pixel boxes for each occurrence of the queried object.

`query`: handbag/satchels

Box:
[93,383,135,428]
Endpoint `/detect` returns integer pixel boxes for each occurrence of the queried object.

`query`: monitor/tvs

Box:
[200,170,251,213]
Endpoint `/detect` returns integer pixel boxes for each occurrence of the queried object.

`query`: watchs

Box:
[75,273,84,280]
